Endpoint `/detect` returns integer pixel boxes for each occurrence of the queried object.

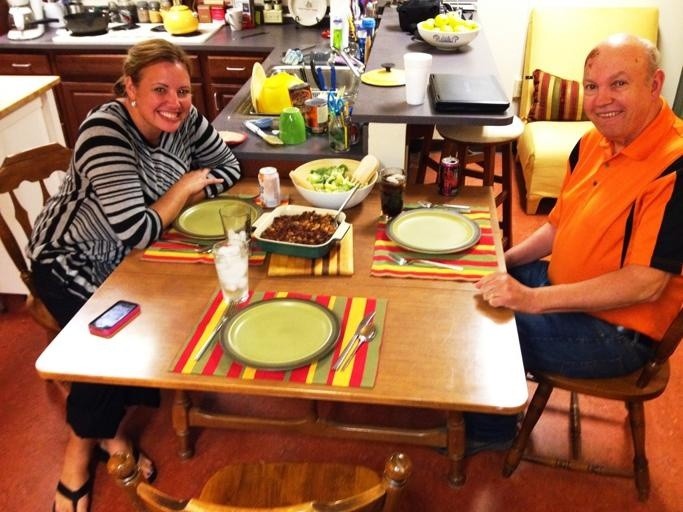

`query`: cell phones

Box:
[88,300,142,336]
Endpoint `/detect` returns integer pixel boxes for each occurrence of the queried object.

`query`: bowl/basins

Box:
[288,158,377,210]
[417,20,480,51]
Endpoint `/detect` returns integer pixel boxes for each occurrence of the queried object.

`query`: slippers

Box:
[132,448,158,484]
[52,478,92,512]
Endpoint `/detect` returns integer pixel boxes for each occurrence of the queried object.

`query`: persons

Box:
[26,40,244,512]
[430,26,683,460]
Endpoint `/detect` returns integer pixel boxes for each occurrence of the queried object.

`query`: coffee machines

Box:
[6,1,44,40]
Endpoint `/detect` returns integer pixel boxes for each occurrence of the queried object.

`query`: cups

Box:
[279,107,305,144]
[224,8,242,31]
[218,202,253,252]
[212,239,249,306]
[379,167,405,222]
[403,52,431,106]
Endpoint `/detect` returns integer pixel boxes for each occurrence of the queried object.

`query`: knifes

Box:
[330,310,377,371]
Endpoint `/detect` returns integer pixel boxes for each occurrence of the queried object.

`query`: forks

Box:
[193,298,240,362]
[384,251,462,273]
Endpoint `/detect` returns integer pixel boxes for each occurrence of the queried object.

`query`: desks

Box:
[0,75,67,296]
[350,4,514,187]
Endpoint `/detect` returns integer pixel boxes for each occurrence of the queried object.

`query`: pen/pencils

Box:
[328,99,352,149]
[241,31,266,38]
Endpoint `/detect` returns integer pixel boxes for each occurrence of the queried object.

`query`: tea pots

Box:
[159,5,198,36]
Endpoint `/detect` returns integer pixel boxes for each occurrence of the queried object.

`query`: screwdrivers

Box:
[282,45,316,57]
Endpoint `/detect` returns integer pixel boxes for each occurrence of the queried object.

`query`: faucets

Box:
[330,44,365,80]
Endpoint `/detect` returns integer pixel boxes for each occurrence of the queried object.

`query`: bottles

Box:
[109,1,159,24]
[328,113,350,153]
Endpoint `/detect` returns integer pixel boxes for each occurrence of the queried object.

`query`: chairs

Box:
[0,141,77,399]
[501,310,681,503]
[106,450,413,512]
[514,7,658,215]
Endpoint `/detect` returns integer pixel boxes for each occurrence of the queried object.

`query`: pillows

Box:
[526,69,593,122]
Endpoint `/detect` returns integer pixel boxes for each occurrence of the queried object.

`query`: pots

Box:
[29,13,109,36]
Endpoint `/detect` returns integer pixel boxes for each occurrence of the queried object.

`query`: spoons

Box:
[340,323,376,372]
[417,200,469,210]
[157,246,213,254]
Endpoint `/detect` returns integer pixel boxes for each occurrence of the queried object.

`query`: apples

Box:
[421,10,477,32]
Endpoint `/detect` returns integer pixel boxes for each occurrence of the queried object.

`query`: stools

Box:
[435,114,524,253]
[415,122,484,189]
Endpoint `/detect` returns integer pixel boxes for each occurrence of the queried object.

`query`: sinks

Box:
[247,91,344,117]
[266,69,353,92]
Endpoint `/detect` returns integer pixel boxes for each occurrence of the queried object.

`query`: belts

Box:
[614,326,654,348]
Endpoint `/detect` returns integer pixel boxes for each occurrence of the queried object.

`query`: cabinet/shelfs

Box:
[204,49,271,124]
[0,50,51,74]
[51,49,203,149]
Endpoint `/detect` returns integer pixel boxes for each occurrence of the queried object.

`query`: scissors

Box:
[327,93,344,115]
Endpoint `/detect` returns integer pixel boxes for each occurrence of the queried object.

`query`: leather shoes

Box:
[438,439,511,457]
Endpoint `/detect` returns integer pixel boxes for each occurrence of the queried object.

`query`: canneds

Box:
[258,166,281,208]
[439,156,460,197]
[289,83,328,134]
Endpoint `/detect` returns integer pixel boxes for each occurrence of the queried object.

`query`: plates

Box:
[287,0,327,26]
[249,62,265,114]
[384,208,481,255]
[217,130,247,146]
[171,196,262,241]
[218,297,342,372]
